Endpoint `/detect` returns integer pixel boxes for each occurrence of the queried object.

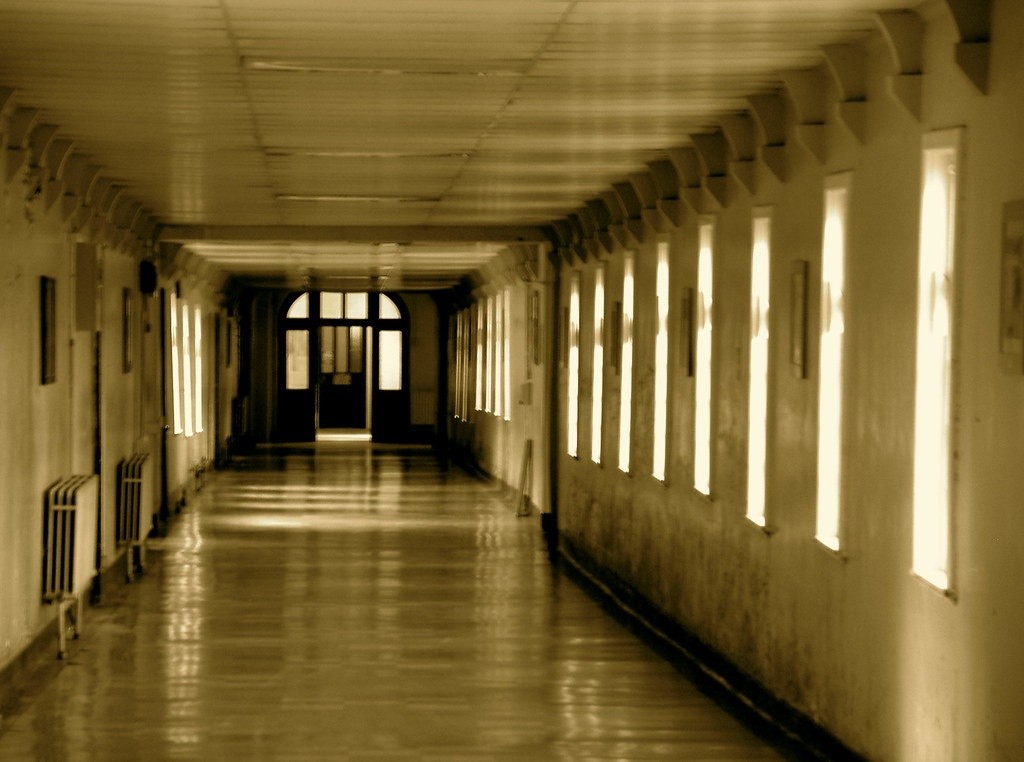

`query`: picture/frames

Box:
[526,290,539,365]
[678,287,693,377]
[788,260,809,379]
[609,301,620,376]
[122,286,133,373]
[40,275,56,385]
[557,307,570,368]
